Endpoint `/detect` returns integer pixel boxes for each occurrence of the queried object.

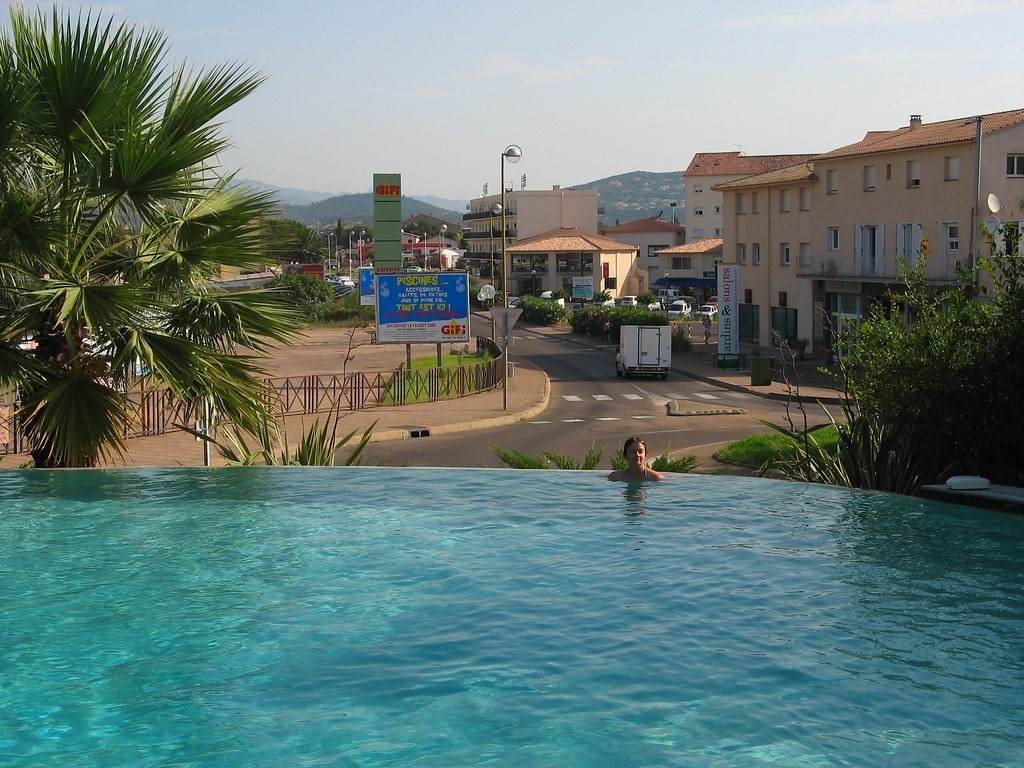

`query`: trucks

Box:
[615,325,672,379]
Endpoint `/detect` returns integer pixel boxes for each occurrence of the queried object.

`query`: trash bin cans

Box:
[748,356,774,387]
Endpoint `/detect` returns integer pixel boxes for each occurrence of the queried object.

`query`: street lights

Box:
[489,201,502,342]
[327,223,448,281]
[964,115,982,295]
[501,144,523,411]
[530,266,536,297]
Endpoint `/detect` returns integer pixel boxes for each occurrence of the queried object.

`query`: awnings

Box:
[652,277,716,288]
[506,274,545,281]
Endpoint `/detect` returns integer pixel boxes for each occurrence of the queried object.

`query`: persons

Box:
[607,437,665,481]
[702,315,712,344]
[603,318,613,345]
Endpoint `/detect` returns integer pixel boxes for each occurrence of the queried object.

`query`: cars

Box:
[705,295,718,306]
[408,266,421,271]
[507,288,696,314]
[695,305,718,318]
[325,274,355,288]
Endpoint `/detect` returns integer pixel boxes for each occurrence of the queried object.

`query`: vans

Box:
[668,300,692,319]
